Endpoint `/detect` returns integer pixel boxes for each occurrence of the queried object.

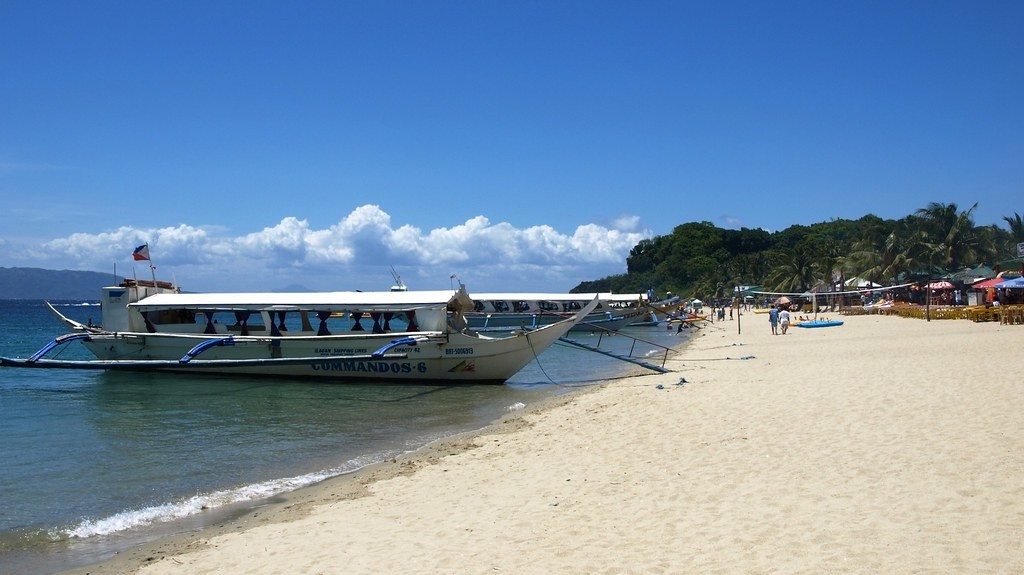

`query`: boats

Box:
[30,260,600,385]
[315,282,709,332]
[787,318,844,328]
[753,307,771,313]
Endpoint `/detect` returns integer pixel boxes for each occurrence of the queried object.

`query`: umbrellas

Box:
[775,297,792,305]
[904,281,956,293]
[799,291,816,301]
[972,276,1024,289]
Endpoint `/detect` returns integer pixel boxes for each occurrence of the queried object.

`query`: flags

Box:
[132,244,151,261]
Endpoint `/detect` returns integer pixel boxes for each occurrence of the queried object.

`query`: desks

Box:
[999,308,1022,325]
[973,309,989,321]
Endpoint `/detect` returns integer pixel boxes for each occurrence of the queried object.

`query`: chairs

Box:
[839,304,1024,325]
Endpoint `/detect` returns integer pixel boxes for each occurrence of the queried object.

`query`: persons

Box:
[590,329,616,336]
[859,289,899,307]
[648,289,677,303]
[940,286,1024,320]
[664,296,844,339]
[477,298,639,313]
